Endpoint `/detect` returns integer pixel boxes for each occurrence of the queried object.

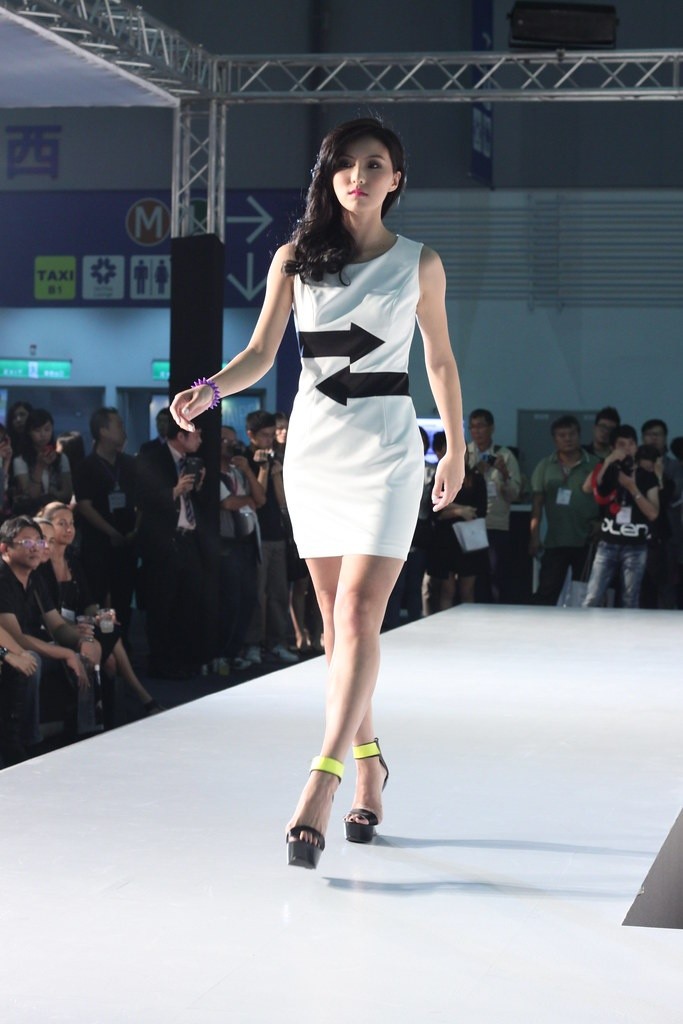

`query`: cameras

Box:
[620,454,635,476]
[486,454,497,467]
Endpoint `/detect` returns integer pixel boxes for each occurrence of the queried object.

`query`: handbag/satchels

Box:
[453,507,488,553]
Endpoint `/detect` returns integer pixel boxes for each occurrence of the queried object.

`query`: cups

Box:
[76,616,94,641]
[96,608,114,633]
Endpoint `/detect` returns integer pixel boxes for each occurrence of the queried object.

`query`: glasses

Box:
[596,423,615,433]
[5,539,45,550]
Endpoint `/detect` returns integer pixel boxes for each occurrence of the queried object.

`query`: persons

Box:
[0,401,312,764]
[409,405,683,616]
[582,425,660,608]
[527,416,600,605]
[168,118,466,873]
[458,409,522,603]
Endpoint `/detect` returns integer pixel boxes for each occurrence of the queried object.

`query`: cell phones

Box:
[40,444,53,456]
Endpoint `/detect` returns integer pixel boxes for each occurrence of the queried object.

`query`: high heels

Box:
[286,757,344,867]
[344,737,389,843]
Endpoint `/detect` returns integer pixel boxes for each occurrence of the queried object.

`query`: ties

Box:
[179,458,194,526]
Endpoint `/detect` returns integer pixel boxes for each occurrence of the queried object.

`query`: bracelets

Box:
[191,377,220,410]
[636,495,640,500]
[31,477,41,483]
[0,648,8,658]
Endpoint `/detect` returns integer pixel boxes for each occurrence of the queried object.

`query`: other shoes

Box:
[230,657,251,670]
[295,629,311,651]
[80,729,103,740]
[265,645,298,663]
[246,648,261,664]
[145,699,168,715]
[104,720,130,731]
[17,740,50,760]
